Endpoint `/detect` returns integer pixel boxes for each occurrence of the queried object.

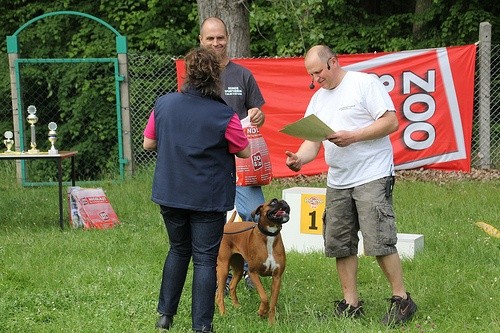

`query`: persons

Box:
[199,18,266,299]
[143,46,250,333]
[286,45,417,328]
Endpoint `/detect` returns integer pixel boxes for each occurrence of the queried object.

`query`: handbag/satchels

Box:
[71,188,121,229]
[235,116,272,186]
[68,187,83,228]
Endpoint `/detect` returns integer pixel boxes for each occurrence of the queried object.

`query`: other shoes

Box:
[156,314,169,330]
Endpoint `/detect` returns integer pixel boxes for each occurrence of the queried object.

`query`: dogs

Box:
[216,199,290,325]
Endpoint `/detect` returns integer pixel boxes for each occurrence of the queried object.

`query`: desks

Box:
[0,151,78,230]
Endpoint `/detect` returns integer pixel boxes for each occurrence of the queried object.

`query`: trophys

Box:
[26,105,39,153]
[48,122,58,155]
[4,131,14,153]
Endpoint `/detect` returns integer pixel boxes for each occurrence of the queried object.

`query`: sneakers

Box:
[336,300,364,317]
[380,292,417,328]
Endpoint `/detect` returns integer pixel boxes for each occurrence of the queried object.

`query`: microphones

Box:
[310,81,314,89]
